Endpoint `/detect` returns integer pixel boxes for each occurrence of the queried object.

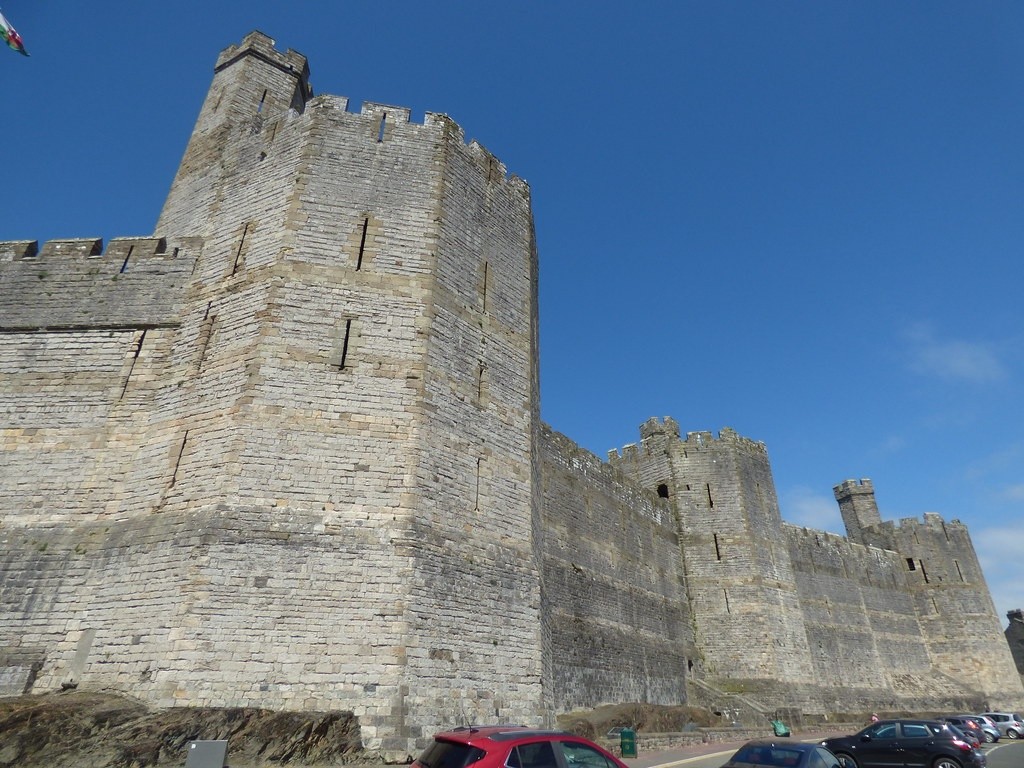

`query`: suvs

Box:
[816,718,987,768]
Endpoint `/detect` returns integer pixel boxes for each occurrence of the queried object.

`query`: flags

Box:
[0,12,31,57]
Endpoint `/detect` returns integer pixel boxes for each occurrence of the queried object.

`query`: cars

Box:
[720,739,846,768]
[975,712,1024,740]
[874,711,1002,749]
[410,725,629,768]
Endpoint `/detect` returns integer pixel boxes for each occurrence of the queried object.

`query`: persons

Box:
[871,712,879,722]
